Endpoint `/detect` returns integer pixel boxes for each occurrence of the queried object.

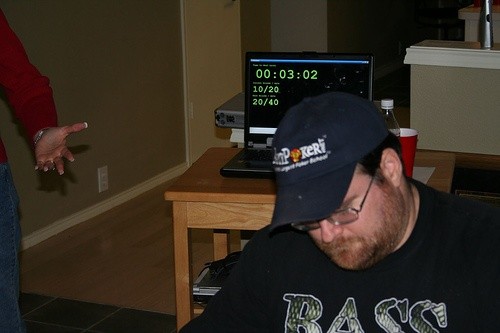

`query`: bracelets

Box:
[34,127,49,147]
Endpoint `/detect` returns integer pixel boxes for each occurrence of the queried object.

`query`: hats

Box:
[269,92,391,231]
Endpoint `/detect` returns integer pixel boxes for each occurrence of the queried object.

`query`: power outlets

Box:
[97,166,109,193]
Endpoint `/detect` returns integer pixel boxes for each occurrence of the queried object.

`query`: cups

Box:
[399,127,418,178]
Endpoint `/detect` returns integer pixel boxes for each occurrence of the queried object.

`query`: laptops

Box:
[218,50,376,178]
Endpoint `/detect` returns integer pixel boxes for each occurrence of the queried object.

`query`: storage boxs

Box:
[404,39,500,155]
[458,4,500,43]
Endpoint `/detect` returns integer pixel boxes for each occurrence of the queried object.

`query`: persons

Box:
[179,92,500,333]
[0,12,88,333]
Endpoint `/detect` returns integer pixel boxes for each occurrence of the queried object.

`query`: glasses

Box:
[291,175,375,232]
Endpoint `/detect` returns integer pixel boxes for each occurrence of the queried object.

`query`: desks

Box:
[163,108,457,333]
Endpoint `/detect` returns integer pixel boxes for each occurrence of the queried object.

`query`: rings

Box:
[45,160,53,163]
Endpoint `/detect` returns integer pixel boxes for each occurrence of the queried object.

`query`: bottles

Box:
[381,99,402,137]
